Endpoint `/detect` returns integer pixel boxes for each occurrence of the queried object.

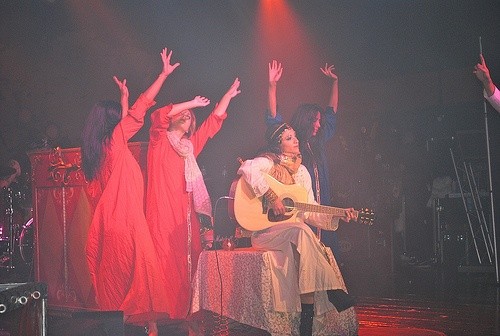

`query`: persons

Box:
[145,77,242,336]
[82,48,180,336]
[474,53,500,111]
[238,122,357,336]
[0,160,20,187]
[265,60,339,267]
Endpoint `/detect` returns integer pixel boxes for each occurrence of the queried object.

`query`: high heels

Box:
[329,290,358,313]
[299,310,314,336]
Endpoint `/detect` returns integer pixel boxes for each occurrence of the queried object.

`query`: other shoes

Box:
[147,327,159,336]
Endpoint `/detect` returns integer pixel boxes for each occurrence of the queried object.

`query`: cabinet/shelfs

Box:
[26,141,150,313]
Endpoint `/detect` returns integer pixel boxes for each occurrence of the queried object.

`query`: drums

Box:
[0,190,28,243]
[20,218,35,264]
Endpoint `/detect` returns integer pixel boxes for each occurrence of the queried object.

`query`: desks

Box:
[0,279,48,336]
[189,246,359,336]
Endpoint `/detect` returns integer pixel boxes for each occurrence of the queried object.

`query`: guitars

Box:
[226,171,375,232]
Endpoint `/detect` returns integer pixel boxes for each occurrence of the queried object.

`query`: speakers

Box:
[47,308,124,336]
